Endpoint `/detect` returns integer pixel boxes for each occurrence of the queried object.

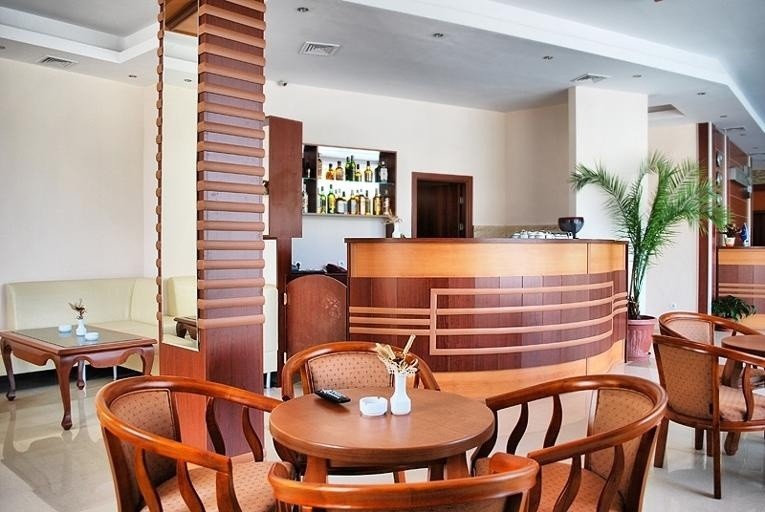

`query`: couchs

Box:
[4,276,160,372]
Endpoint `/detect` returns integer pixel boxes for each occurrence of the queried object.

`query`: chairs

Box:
[650,310,764,500]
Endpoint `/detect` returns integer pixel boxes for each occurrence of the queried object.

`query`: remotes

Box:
[315,389,351,403]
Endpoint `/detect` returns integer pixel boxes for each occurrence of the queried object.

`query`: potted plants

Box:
[564,148,741,360]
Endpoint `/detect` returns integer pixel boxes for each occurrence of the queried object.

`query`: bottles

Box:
[316,185,392,217]
[302,184,308,213]
[740,221,748,241]
[304,161,312,178]
[315,152,390,184]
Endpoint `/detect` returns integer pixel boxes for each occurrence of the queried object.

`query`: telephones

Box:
[325,264,348,273]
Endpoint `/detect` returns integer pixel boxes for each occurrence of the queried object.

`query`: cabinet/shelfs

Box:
[301,138,399,219]
[727,137,748,243]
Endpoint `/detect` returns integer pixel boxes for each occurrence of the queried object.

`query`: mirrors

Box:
[160,0,200,351]
